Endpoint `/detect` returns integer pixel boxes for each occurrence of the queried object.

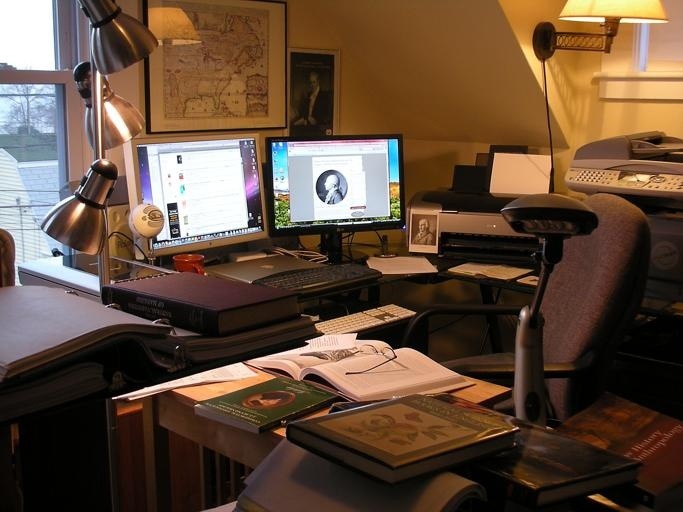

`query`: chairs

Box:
[400,193,651,431]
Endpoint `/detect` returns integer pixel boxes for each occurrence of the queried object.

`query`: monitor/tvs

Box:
[122,134,269,264]
[265,134,406,266]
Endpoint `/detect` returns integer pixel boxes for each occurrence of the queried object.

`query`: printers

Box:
[564,131,683,303]
[407,145,555,277]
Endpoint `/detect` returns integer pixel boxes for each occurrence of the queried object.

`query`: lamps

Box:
[38,0,159,285]
[532,0,671,62]
[501,194,598,426]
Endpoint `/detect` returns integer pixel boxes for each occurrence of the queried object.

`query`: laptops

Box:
[204,255,329,285]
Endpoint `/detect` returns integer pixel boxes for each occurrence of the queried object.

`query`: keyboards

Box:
[252,263,384,291]
[314,304,417,336]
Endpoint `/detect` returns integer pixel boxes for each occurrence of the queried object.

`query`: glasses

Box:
[337,345,397,374]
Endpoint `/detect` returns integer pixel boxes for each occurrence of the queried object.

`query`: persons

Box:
[323,173,346,204]
[291,59,333,135]
[411,219,435,246]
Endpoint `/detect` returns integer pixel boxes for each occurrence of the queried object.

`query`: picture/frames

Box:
[140,0,340,140]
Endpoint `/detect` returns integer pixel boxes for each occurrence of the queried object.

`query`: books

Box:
[101,270,301,336]
[0,354,109,426]
[110,334,681,512]
[0,285,316,384]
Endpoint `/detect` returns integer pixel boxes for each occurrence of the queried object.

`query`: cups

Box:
[172,252,205,275]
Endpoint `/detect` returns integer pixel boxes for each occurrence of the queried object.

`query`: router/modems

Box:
[227,250,268,263]
